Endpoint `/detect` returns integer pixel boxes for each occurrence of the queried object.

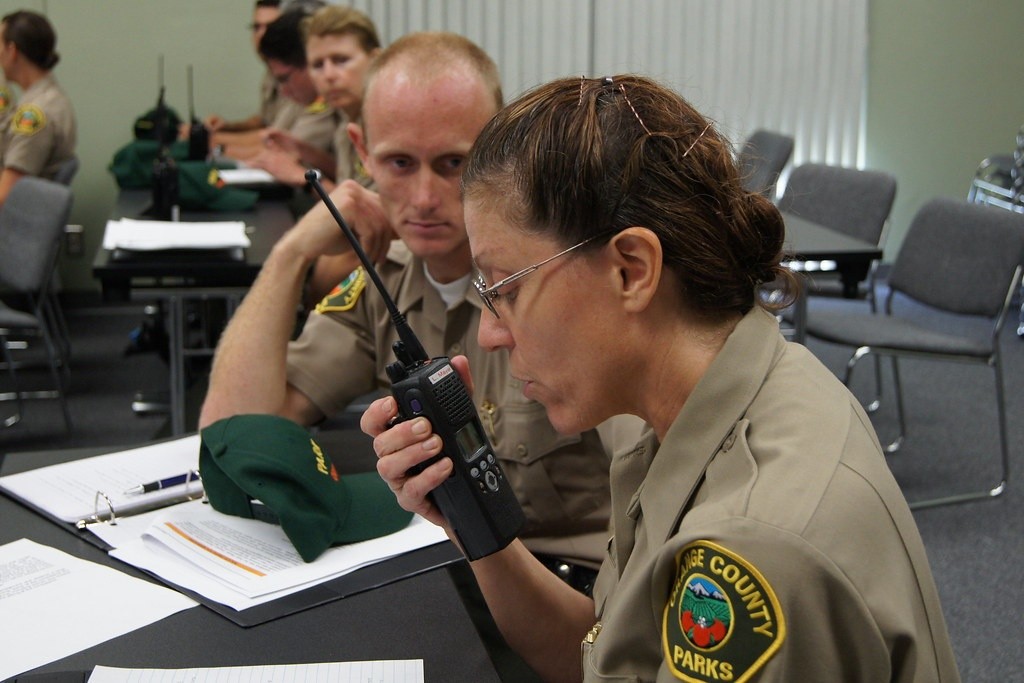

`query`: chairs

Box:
[0,178,80,432]
[735,128,1024,512]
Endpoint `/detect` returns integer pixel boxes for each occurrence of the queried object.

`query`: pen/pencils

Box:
[580,623,602,673]
[125,470,200,496]
[479,399,497,435]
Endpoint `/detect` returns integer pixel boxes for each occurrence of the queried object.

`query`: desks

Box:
[0,427,587,683]
[93,188,295,436]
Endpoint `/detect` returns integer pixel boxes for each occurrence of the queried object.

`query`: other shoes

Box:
[123,317,173,357]
[132,356,205,413]
[178,300,227,346]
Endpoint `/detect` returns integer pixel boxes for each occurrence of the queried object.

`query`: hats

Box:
[151,161,259,215]
[106,140,177,192]
[199,414,415,564]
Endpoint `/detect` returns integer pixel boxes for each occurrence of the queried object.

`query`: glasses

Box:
[275,66,297,91]
[469,228,684,321]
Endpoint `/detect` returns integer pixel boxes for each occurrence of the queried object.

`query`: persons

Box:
[0,11,76,208]
[360,74,961,683]
[179,0,384,288]
[198,30,651,683]
